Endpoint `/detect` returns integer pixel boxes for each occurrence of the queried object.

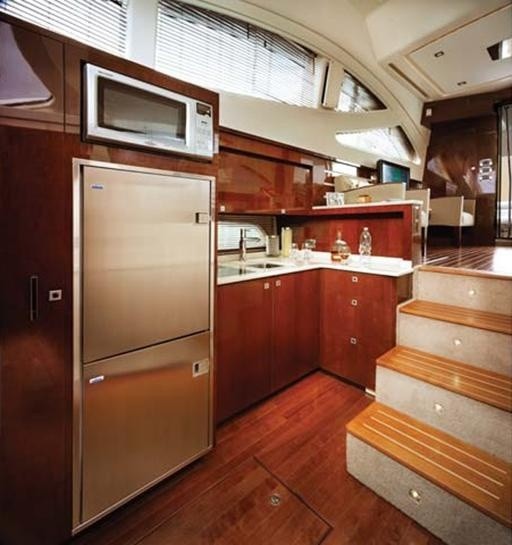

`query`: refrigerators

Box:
[71,155,215,538]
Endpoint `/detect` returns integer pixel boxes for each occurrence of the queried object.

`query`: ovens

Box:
[81,63,215,163]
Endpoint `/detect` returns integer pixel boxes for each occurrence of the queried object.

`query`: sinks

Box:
[217,264,256,279]
[243,262,283,270]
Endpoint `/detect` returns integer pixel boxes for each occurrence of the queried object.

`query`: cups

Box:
[266,226,293,257]
[338,245,351,265]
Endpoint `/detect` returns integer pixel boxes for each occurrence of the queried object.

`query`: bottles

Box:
[358,227,372,268]
[332,229,347,263]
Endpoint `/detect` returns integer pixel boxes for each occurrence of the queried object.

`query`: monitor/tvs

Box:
[376,159,410,191]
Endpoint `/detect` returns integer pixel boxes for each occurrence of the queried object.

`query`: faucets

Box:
[239,228,261,262]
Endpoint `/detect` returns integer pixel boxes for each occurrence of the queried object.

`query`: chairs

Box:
[334,174,478,262]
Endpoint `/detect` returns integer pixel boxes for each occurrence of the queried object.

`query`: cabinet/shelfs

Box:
[213,268,319,428]
[0,20,74,543]
[318,268,412,393]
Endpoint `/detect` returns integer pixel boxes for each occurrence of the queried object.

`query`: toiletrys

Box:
[287,239,319,267]
[266,234,280,256]
